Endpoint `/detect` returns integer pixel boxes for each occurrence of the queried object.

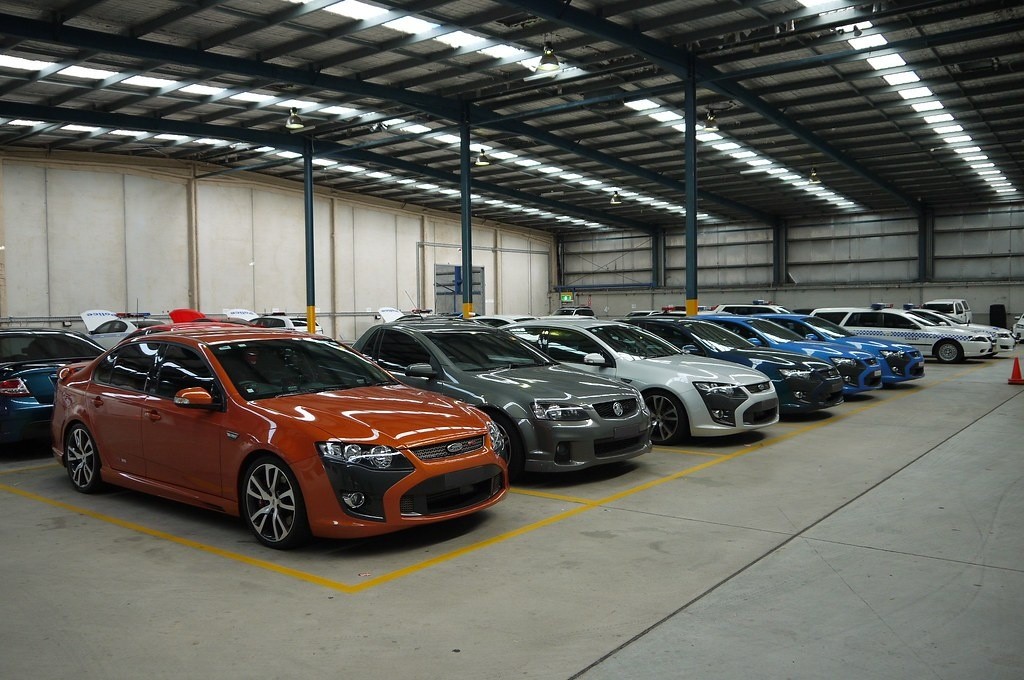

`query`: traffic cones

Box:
[1006,356,1024,385]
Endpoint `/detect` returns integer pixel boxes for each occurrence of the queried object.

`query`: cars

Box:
[500,318,781,446]
[625,297,1024,395]
[47,328,511,550]
[610,314,845,415]
[79,309,325,350]
[0,326,108,445]
[341,315,653,485]
[377,303,595,334]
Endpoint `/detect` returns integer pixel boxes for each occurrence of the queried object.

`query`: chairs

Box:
[26,337,59,358]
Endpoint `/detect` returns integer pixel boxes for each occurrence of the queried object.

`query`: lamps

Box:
[808,168,823,185]
[610,191,622,205]
[285,107,305,129]
[538,32,560,70]
[475,148,490,166]
[703,110,720,131]
[853,25,863,37]
